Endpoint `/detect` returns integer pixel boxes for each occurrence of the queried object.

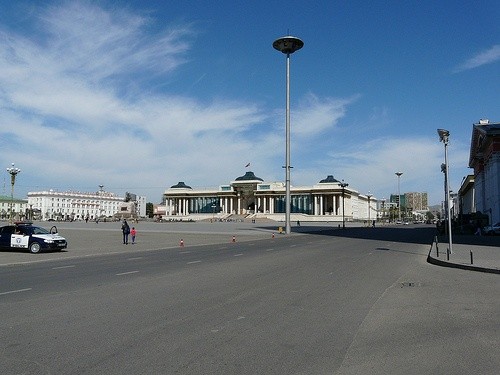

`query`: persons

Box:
[252,219,256,224]
[153,214,163,222]
[436,218,442,231]
[130,227,137,244]
[372,220,376,233]
[85,215,107,224]
[474,220,482,236]
[297,220,300,227]
[121,221,130,245]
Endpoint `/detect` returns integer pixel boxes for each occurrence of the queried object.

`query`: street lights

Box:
[394,169,403,222]
[365,191,387,225]
[6,162,21,225]
[211,204,217,218]
[273,28,304,234]
[338,179,349,229]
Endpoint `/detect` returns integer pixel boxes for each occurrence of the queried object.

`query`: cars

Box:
[483,222,500,236]
[396,220,424,225]
[0,221,67,254]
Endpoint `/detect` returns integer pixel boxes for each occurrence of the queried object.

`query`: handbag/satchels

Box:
[128,228,130,234]
